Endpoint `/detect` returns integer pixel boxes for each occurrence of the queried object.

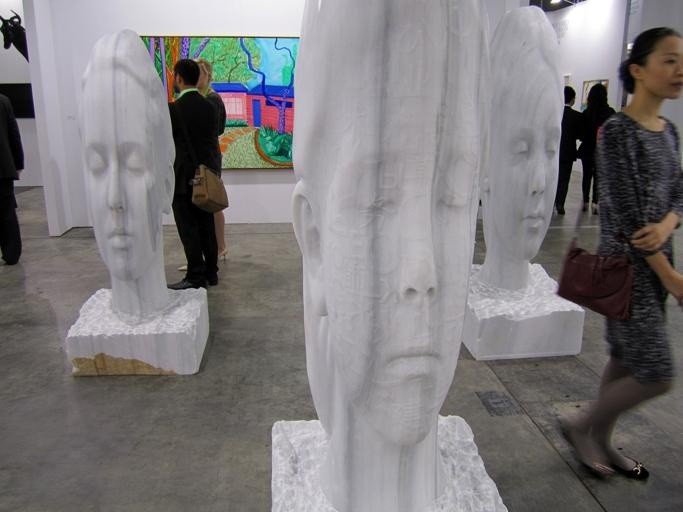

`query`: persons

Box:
[72,27,180,326]
[576,83,616,217]
[551,27,682,483]
[290,1,489,501]
[190,55,228,264]
[481,5,565,295]
[0,94,25,267]
[554,85,579,214]
[167,59,219,291]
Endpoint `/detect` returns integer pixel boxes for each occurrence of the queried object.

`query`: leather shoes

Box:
[555,206,565,215]
[205,277,217,286]
[559,419,614,480]
[166,277,206,289]
[590,203,598,215]
[582,202,589,212]
[612,454,649,480]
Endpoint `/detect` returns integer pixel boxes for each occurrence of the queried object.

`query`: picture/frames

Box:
[137,35,300,171]
[562,73,608,108]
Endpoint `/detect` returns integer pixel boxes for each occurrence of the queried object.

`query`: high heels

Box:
[177,265,187,271]
[218,248,227,262]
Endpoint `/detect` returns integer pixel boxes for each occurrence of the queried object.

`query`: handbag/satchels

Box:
[190,164,228,214]
[556,245,635,321]
